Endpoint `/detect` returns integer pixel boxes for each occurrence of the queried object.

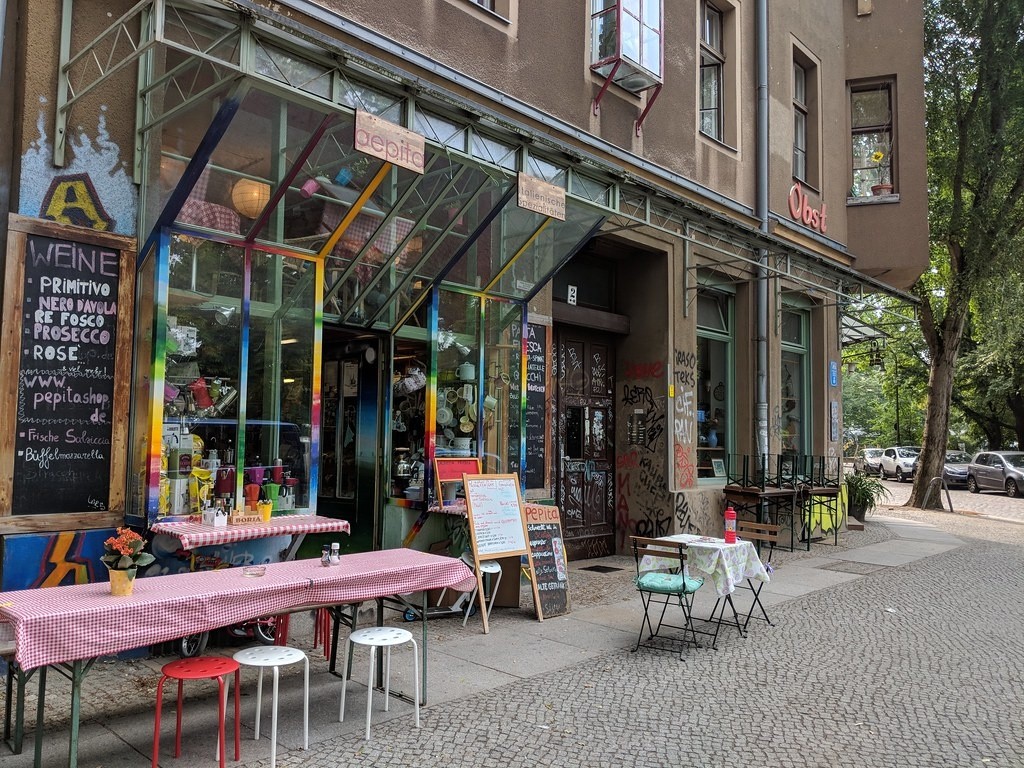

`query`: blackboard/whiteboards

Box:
[464,474,530,559]
[503,312,552,501]
[525,522,572,618]
[0,212,138,533]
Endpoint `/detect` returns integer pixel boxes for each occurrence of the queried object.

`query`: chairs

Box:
[706,521,780,633]
[629,535,702,661]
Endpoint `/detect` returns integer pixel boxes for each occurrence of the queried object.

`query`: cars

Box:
[910,450,973,486]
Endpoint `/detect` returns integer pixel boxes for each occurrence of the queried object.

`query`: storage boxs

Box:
[162,423,193,517]
[168,315,198,352]
[428,539,522,608]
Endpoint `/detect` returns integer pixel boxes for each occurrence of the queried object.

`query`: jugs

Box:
[448,437,472,458]
[244,468,281,509]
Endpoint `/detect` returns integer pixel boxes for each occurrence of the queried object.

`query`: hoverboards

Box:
[401,592,478,622]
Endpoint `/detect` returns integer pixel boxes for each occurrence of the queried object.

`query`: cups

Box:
[257,504,272,521]
[483,395,497,410]
[455,362,475,379]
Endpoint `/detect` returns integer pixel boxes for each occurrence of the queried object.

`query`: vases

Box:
[110,570,135,596]
[870,183,892,196]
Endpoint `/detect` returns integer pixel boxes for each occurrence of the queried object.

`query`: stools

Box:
[214,646,308,768]
[437,551,502,627]
[277,607,330,659]
[338,626,420,740]
[152,657,240,768]
[720,453,840,494]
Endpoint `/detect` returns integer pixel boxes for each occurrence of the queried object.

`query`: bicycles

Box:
[169,548,280,659]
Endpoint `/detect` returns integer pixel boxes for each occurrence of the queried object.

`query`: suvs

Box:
[965,450,1024,499]
[852,447,886,479]
[878,445,922,483]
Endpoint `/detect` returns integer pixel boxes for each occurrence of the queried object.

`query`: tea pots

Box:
[403,486,420,499]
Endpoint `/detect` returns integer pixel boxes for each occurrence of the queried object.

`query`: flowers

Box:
[100,524,158,580]
[871,150,889,185]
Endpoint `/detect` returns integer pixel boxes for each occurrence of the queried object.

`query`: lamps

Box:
[213,304,237,326]
[868,338,887,373]
[841,357,859,377]
[233,176,271,219]
[453,341,473,359]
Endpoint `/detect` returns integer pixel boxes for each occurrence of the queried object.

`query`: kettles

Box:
[279,484,296,509]
[392,447,417,477]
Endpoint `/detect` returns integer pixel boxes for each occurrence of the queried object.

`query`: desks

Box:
[0,548,475,768]
[640,533,770,652]
[725,490,843,558]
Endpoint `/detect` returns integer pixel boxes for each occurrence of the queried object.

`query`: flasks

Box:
[273,458,282,483]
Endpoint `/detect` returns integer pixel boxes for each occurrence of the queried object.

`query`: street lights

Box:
[877,349,902,447]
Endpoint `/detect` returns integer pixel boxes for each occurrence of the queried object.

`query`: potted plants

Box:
[605,18,648,88]
[446,201,464,227]
[300,170,331,199]
[334,158,371,187]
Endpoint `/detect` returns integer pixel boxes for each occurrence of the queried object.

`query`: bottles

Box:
[724,507,737,543]
[321,545,331,567]
[331,542,340,565]
